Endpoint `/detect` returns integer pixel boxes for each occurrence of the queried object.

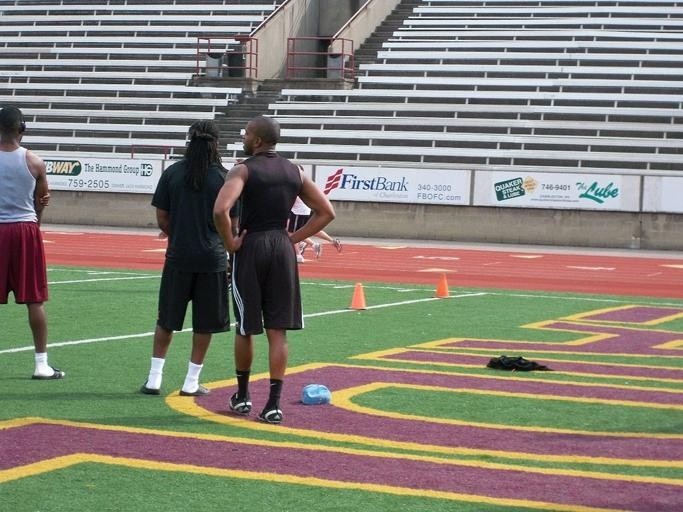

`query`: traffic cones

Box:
[346,282,369,311]
[431,270,452,299]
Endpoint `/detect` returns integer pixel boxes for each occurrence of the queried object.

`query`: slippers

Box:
[32,367,66,379]
[141,380,160,395]
[180,384,210,396]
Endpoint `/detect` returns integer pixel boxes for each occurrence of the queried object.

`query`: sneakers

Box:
[312,243,320,259]
[333,239,342,253]
[230,392,252,415]
[297,254,304,263]
[257,408,283,423]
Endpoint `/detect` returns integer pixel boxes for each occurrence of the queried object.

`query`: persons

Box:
[139,120,242,397]
[0,107,66,380]
[212,116,336,427]
[286,196,341,262]
